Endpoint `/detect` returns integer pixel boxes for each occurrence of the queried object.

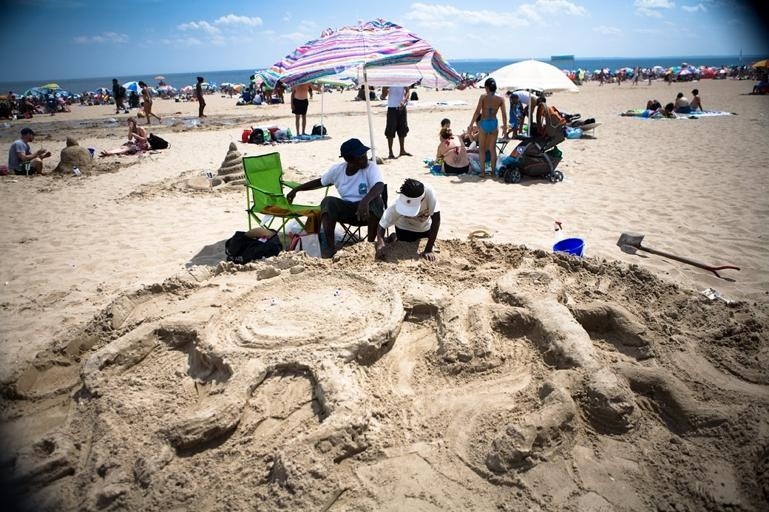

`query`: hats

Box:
[337,137,374,159]
[21,127,38,137]
[395,177,426,218]
[485,77,497,87]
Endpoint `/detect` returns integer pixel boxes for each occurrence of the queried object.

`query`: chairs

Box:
[460,103,602,183]
[241,153,332,251]
[336,182,390,252]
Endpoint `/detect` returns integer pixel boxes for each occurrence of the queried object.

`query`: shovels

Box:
[616,233,741,282]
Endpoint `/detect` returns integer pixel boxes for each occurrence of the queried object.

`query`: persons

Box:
[287,138,386,258]
[376,179,441,262]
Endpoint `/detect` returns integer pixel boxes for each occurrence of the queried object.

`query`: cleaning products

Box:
[552,221,564,250]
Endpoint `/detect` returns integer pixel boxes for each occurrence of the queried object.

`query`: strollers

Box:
[498,102,567,185]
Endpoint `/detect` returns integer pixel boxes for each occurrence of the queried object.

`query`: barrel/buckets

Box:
[551,238,585,259]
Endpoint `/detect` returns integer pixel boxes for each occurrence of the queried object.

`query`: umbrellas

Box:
[254,19,463,166]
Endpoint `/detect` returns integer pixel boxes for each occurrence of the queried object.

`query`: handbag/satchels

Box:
[225,225,283,265]
[290,230,322,261]
[241,126,288,144]
[410,91,419,100]
[312,123,327,135]
[147,132,169,149]
